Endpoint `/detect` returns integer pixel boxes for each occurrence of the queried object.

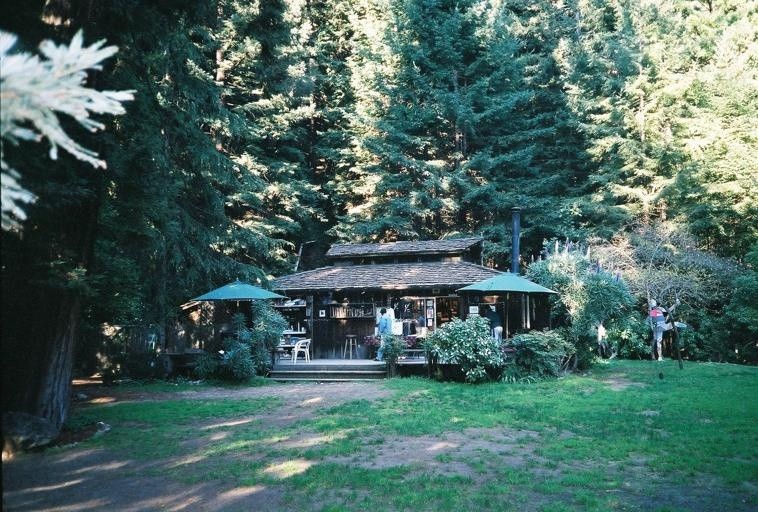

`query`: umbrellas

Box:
[191,277,289,342]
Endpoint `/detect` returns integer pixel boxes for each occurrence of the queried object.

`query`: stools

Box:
[343,335,359,359]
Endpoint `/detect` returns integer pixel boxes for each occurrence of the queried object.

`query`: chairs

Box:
[291,339,312,364]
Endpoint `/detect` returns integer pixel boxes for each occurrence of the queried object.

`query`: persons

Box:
[373,308,392,361]
[485,308,503,344]
[416,311,425,327]
[645,298,687,361]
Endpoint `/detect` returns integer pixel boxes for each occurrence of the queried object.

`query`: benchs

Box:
[399,349,424,359]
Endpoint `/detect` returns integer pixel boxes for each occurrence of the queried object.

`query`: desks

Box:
[363,335,416,359]
[275,345,307,359]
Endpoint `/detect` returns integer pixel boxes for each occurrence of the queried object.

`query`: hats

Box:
[380,308,386,313]
